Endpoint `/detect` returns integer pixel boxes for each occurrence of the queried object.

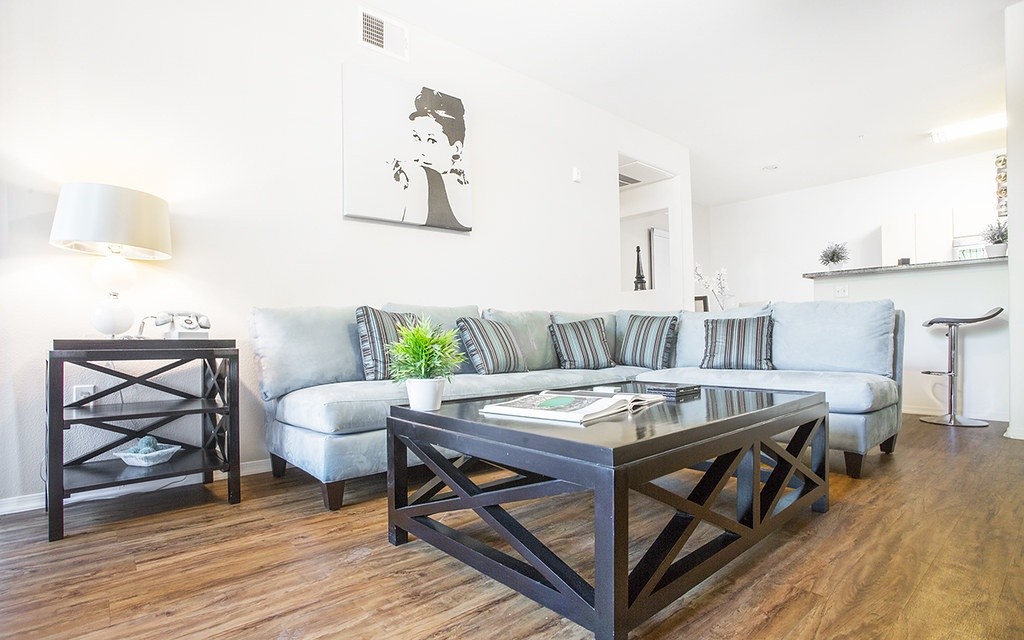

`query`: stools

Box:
[918,306,1004,429]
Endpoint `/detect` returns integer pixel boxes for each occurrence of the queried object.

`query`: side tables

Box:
[46,339,240,544]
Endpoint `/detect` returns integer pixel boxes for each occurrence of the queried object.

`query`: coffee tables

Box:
[384,379,831,640]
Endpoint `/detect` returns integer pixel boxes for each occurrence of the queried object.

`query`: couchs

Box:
[253,297,905,513]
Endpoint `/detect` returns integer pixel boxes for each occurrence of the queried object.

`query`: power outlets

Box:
[72,387,95,407]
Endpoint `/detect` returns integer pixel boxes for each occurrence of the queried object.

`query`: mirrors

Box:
[651,227,669,290]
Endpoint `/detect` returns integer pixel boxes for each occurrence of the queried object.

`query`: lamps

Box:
[48,182,173,337]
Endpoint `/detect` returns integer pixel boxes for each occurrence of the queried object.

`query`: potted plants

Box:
[984,220,1008,258]
[387,317,465,411]
[819,242,849,270]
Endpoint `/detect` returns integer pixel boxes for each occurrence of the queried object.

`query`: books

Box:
[483,390,666,422]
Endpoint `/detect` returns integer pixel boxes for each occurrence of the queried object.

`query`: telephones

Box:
[154,312,211,339]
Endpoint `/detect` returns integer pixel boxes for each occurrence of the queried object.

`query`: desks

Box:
[799,257,1011,423]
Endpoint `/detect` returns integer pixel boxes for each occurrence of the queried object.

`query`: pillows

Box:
[546,317,613,368]
[354,306,420,381]
[699,315,776,369]
[617,312,678,371]
[457,317,528,374]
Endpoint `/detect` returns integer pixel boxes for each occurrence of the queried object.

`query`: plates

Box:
[113,443,182,467]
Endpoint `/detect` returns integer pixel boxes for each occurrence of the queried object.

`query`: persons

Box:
[390,88,472,230]
